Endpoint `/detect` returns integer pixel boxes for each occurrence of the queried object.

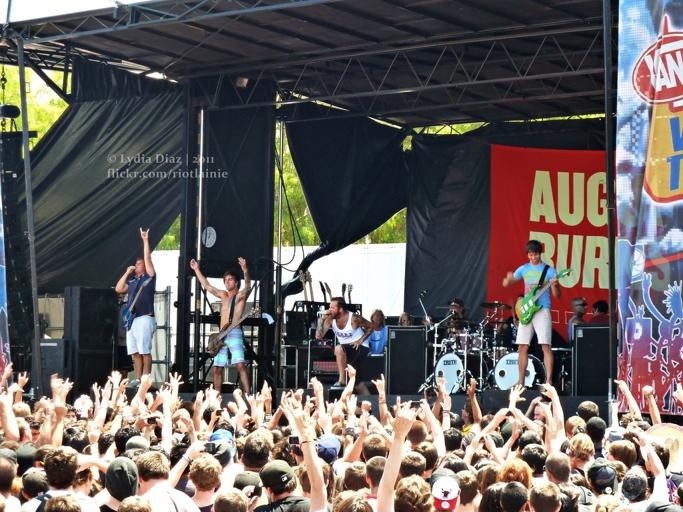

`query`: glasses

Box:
[573,304,587,308]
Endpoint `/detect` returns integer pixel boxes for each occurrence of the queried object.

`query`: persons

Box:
[113,226,157,386]
[502,239,560,387]
[314,297,374,395]
[190,256,252,393]
[0,361,683,512]
[369,295,609,354]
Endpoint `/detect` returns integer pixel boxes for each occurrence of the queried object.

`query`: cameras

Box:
[287,435,300,446]
[248,487,262,498]
[410,401,423,408]
[427,387,439,396]
[216,409,224,416]
[147,416,160,425]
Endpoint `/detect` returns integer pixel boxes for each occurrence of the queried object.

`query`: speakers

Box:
[62,287,118,407]
[385,325,428,395]
[0,131,39,393]
[31,339,74,407]
[571,324,609,397]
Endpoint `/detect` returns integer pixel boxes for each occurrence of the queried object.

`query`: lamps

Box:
[229,71,253,91]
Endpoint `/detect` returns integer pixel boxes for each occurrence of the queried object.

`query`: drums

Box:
[491,346,512,362]
[434,351,488,394]
[456,334,482,351]
[494,352,546,389]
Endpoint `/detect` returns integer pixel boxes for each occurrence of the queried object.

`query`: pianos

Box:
[185,315,267,326]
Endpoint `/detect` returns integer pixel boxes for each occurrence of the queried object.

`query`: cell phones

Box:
[200,442,215,454]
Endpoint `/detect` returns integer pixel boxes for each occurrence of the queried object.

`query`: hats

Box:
[449,298,465,315]
[106,423,683,511]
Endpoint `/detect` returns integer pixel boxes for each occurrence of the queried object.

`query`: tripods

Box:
[416,315,452,393]
[450,333,480,396]
[475,314,495,392]
[486,318,498,390]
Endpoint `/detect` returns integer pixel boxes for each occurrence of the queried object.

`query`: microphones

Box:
[317,312,332,316]
[420,289,428,298]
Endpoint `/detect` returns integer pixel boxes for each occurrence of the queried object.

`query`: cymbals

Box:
[481,302,512,310]
[434,305,470,309]
[490,320,511,323]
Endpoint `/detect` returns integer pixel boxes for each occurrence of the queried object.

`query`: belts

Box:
[146,314,155,317]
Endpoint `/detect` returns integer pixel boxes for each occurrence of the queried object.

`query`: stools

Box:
[551,347,572,396]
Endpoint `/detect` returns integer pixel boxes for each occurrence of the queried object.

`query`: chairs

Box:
[307,328,347,392]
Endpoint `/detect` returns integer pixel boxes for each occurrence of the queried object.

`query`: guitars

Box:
[123,278,154,331]
[207,307,262,357]
[299,269,352,346]
[515,269,572,325]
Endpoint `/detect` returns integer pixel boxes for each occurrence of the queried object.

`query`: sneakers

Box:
[124,377,142,388]
[329,381,345,390]
